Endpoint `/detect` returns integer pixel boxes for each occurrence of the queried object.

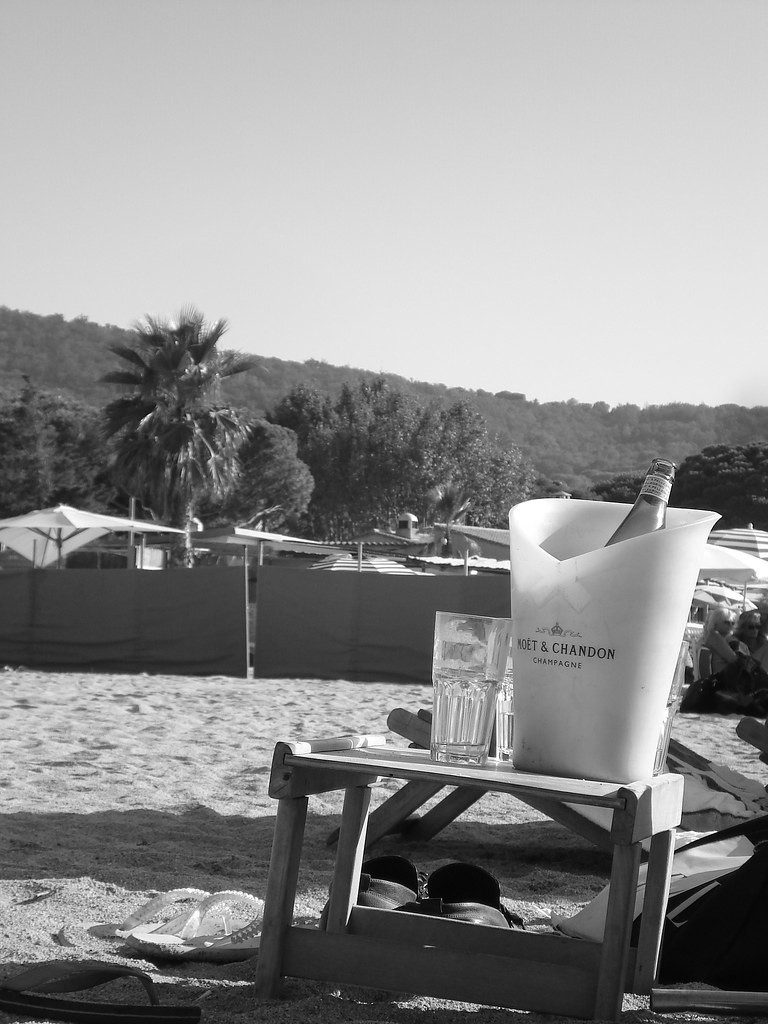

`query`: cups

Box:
[494,637,513,763]
[430,609,513,768]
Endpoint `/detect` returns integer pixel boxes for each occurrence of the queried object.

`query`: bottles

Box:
[604,458,677,545]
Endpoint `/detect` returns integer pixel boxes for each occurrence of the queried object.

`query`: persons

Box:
[680,602,768,716]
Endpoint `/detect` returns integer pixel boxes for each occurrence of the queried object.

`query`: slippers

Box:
[125,890,264,960]
[0,960,201,1024]
[58,888,249,958]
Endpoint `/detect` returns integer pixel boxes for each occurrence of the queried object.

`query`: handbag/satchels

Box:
[681,656,768,718]
[555,816,768,994]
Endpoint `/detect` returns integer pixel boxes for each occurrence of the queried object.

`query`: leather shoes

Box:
[316,855,428,930]
[404,862,527,931]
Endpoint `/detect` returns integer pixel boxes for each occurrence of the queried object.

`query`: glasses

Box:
[748,625,762,631]
[724,620,735,626]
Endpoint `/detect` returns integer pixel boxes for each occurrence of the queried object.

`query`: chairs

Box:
[325,707,767,865]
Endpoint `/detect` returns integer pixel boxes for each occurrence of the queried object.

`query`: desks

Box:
[253,734,685,1024]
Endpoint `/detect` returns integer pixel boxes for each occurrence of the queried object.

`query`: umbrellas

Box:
[691,522,768,610]
[0,504,185,569]
[307,554,412,573]
